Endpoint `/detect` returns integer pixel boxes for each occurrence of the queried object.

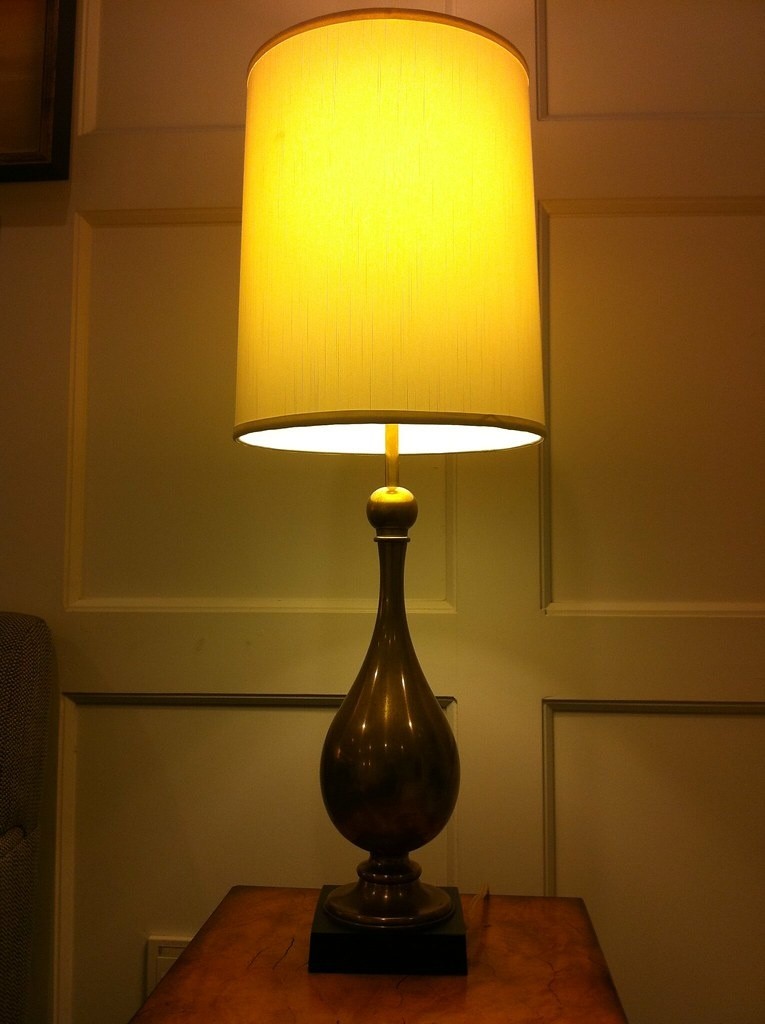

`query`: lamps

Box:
[234,7,539,978]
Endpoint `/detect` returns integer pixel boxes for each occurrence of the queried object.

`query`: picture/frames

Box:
[0,0,77,182]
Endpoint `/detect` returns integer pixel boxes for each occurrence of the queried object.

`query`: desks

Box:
[131,885,631,1024]
